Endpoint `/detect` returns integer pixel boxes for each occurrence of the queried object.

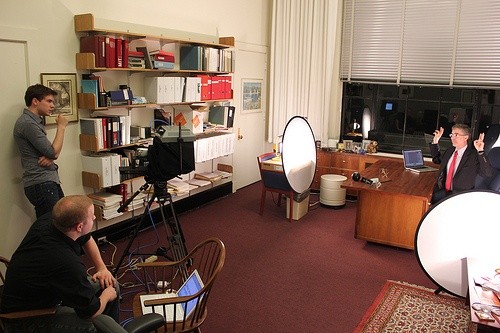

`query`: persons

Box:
[428,123,494,204]
[13,83,69,219]
[378,96,414,135]
[0,194,120,328]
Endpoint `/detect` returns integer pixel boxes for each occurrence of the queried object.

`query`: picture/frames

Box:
[40,73,78,126]
[398,86,415,98]
[240,78,264,114]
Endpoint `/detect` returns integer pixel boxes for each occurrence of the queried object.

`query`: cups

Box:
[479,291,494,318]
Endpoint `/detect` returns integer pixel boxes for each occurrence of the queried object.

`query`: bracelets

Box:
[477,151,485,153]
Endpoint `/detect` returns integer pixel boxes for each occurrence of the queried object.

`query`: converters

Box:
[157,281,167,288]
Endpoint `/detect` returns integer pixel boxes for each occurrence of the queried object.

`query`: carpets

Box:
[354,281,477,333]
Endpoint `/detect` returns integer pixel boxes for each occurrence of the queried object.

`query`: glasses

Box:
[449,134,466,137]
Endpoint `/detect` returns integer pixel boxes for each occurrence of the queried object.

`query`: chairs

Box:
[0,257,56,333]
[132,238,226,333]
[257,153,293,223]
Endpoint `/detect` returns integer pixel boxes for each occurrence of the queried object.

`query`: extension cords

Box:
[145,255,158,263]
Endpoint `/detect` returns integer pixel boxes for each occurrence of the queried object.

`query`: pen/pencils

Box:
[381,180,392,183]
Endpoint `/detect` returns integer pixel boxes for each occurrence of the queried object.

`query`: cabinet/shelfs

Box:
[310,151,358,198]
[75,14,236,222]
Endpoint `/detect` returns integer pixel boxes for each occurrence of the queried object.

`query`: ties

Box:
[446,151,458,191]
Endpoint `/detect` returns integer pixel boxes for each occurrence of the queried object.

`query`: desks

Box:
[341,160,439,257]
[466,257,500,333]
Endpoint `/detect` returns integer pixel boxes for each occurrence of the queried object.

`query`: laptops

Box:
[402,149,439,173]
[141,269,211,323]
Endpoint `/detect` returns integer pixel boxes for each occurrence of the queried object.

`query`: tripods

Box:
[114,190,189,283]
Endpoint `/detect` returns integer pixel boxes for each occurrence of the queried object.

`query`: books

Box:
[145,75,233,104]
[80,34,176,70]
[81,74,107,106]
[179,46,234,73]
[80,114,236,221]
